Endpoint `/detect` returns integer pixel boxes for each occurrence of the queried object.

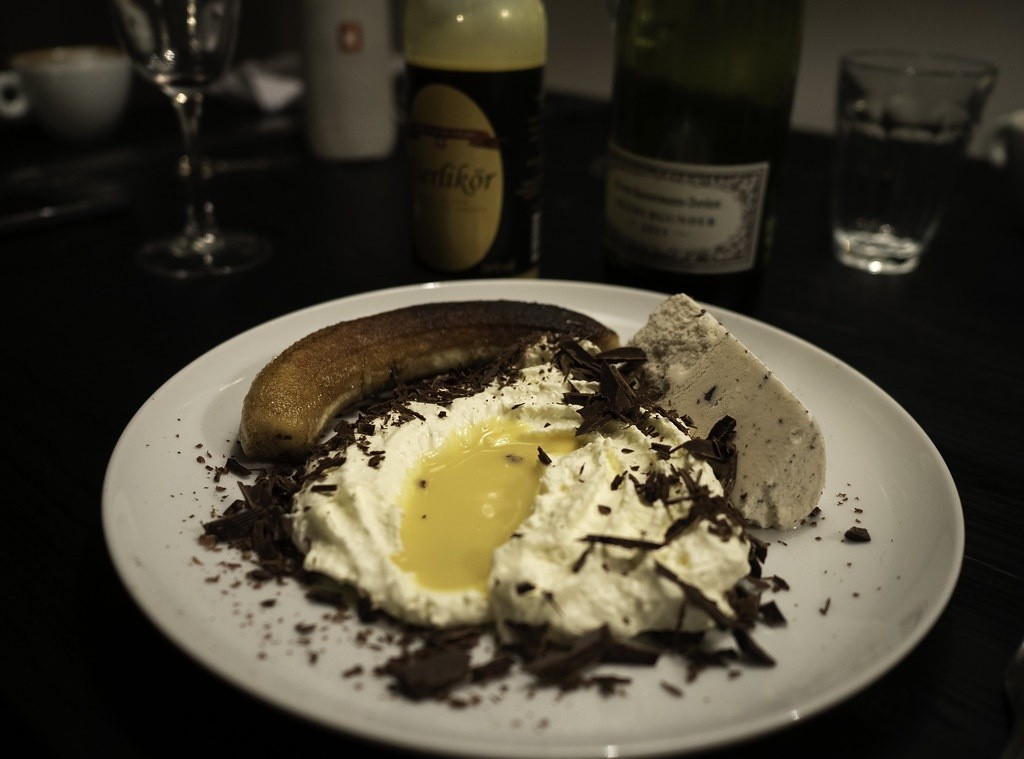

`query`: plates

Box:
[92,279,972,759]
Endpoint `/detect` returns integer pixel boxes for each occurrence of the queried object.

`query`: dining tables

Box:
[0,90,1023,759]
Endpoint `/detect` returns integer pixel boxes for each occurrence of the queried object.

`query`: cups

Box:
[1,48,134,139]
[830,49,998,277]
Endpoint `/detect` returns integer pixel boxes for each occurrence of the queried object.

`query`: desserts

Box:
[202,293,829,705]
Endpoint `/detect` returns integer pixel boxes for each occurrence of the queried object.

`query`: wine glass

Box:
[115,1,274,277]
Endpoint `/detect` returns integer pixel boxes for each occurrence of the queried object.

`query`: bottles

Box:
[599,1,807,314]
[298,0,402,161]
[401,1,549,281]
